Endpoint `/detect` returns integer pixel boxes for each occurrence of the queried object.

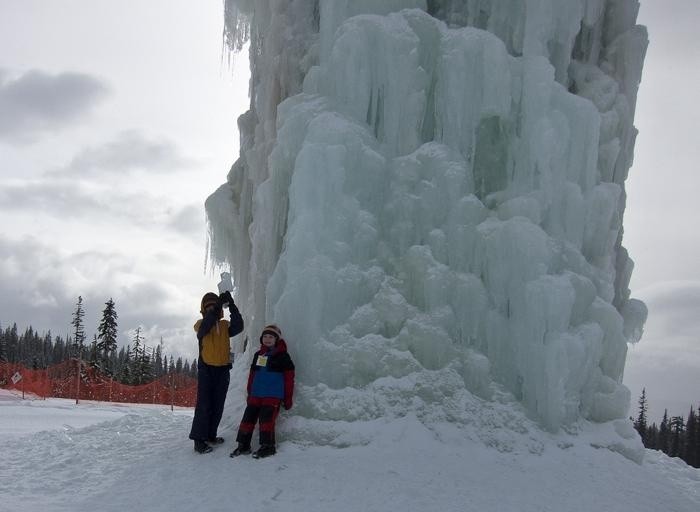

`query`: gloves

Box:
[216,290,234,306]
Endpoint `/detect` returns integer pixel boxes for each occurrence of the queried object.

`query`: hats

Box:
[201,292,222,311]
[262,324,281,338]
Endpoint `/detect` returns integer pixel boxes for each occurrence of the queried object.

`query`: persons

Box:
[228,323,294,458]
[188,291,244,456]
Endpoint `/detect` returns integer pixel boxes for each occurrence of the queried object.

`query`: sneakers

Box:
[206,437,224,445]
[194,442,213,454]
[230,446,252,458]
[252,445,277,459]
